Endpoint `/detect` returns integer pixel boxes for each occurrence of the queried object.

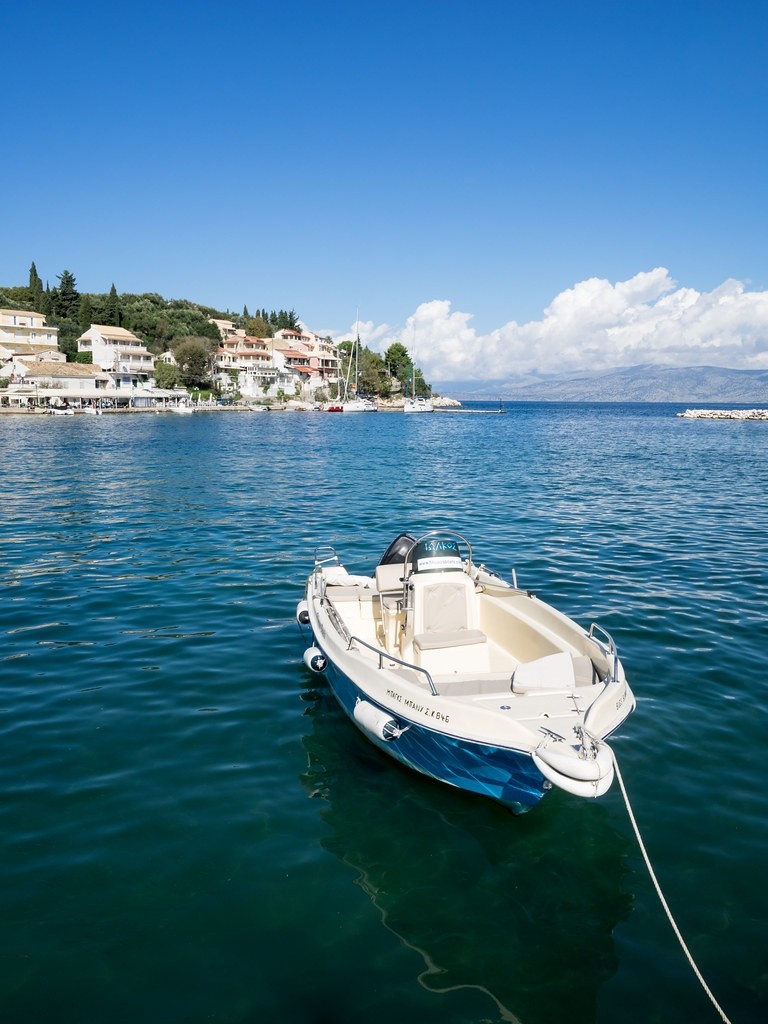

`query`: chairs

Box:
[407,569,490,683]
[375,562,411,656]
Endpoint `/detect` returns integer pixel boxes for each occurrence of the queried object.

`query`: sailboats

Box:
[404,316,434,413]
[342,307,377,412]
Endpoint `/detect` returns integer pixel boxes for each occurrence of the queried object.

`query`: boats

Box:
[85,407,102,415]
[321,404,343,412]
[249,406,270,411]
[171,407,200,413]
[296,529,638,817]
